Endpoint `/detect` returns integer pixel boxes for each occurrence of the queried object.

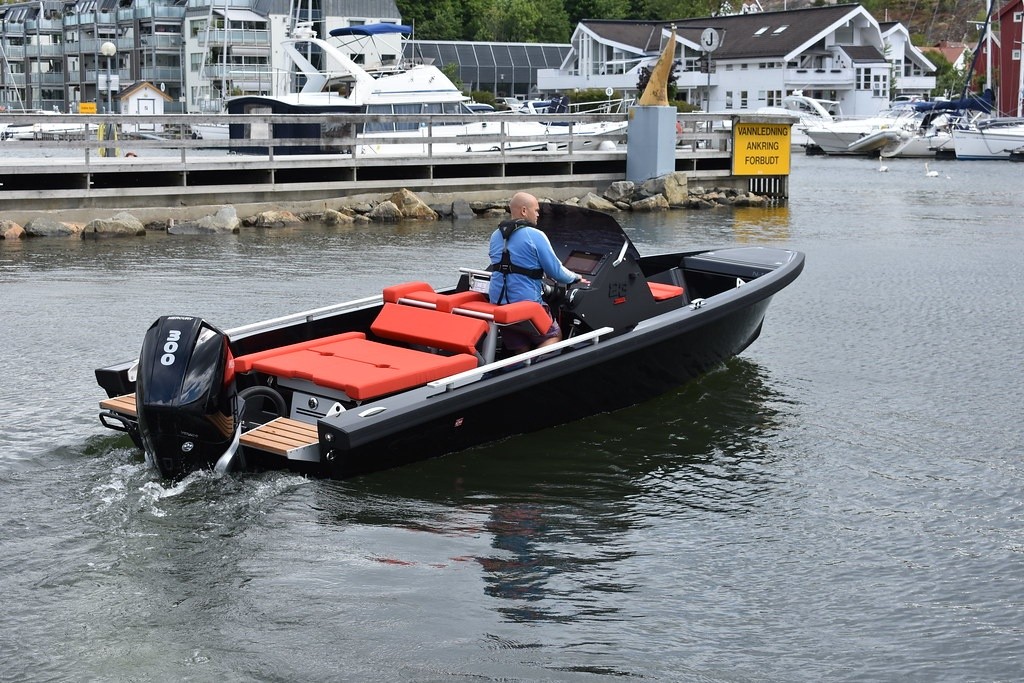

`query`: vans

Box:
[491,97,522,111]
[889,95,924,109]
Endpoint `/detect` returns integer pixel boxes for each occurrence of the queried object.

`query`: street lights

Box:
[101,41,117,114]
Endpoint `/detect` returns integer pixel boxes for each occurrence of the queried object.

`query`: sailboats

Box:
[949,0,1024,162]
[880,0,1011,158]
[188,0,274,141]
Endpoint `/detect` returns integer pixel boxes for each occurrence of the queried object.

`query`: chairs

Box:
[135,314,287,439]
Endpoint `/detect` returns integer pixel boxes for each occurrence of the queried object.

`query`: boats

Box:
[227,22,629,156]
[722,85,845,152]
[94,201,807,486]
[799,93,895,158]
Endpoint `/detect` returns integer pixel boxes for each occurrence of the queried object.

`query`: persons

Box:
[676,121,682,135]
[488,192,590,359]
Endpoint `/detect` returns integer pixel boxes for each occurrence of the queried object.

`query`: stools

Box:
[383,281,553,364]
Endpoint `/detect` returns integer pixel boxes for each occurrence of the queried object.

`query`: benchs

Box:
[647,281,683,313]
